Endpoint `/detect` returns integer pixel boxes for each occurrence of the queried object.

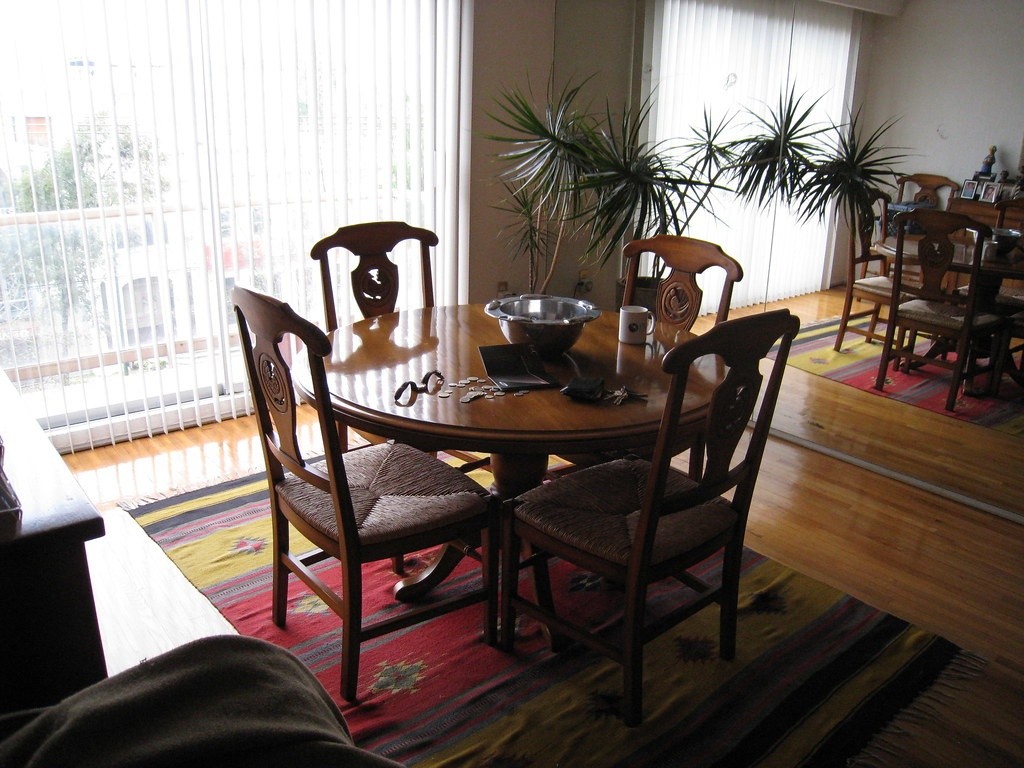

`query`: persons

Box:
[998,171,1009,183]
[981,145,997,172]
[963,183,974,197]
[983,187,994,199]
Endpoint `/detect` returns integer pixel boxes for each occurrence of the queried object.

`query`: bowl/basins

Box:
[966,228,1024,256]
[483,294,602,356]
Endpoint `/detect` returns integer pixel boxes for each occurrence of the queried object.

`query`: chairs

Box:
[556,234,744,482]
[836,174,1024,411]
[498,308,801,729]
[311,222,491,473]
[231,286,500,704]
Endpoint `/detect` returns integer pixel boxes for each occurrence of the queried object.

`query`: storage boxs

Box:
[881,199,936,238]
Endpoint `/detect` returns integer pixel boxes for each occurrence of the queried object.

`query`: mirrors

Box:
[543,0,1024,528]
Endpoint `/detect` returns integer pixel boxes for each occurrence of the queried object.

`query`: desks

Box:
[872,232,1024,397]
[0,368,107,713]
[291,304,730,654]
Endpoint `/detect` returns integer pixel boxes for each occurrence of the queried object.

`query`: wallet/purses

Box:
[561,376,606,401]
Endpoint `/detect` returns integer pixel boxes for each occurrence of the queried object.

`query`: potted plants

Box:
[498,77,706,321]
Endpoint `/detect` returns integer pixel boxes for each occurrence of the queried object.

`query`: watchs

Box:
[394,371,444,401]
[394,379,444,407]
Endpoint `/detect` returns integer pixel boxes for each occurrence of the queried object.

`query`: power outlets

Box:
[578,268,590,297]
[497,282,509,300]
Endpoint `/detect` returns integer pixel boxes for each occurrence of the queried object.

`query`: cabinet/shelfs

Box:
[941,197,1024,296]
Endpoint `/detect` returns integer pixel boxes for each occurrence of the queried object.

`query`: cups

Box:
[981,241,998,261]
[618,306,655,344]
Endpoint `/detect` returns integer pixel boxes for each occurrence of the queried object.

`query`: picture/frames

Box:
[959,179,979,201]
[971,171,997,200]
[978,181,1002,203]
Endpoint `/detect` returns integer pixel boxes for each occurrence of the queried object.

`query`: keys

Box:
[604,390,628,405]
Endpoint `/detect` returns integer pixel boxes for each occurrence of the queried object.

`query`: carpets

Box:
[763,303,1024,441]
[116,439,989,768]
